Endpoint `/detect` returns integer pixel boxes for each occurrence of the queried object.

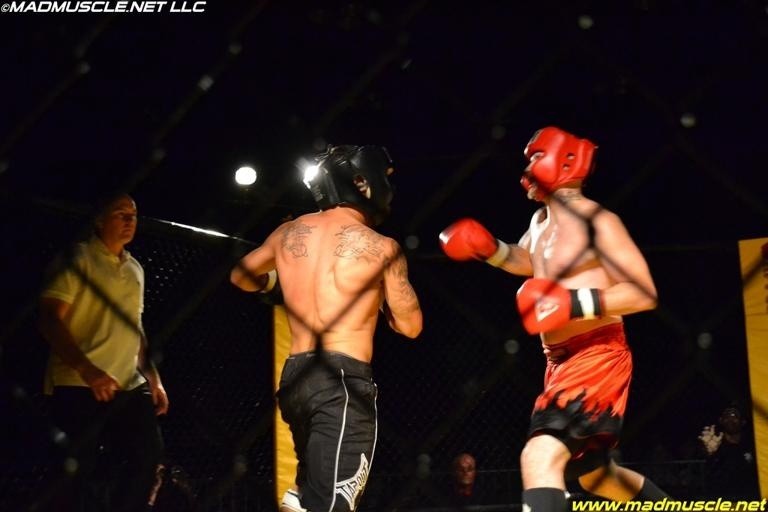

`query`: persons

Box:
[432,454,504,507]
[439,125,681,512]
[231,144,423,512]
[35,191,170,512]
[698,407,760,502]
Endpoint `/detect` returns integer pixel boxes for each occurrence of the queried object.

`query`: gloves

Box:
[515,278,603,335]
[439,219,510,269]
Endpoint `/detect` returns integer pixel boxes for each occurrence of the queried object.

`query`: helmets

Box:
[524,125,609,202]
[310,143,395,228]
